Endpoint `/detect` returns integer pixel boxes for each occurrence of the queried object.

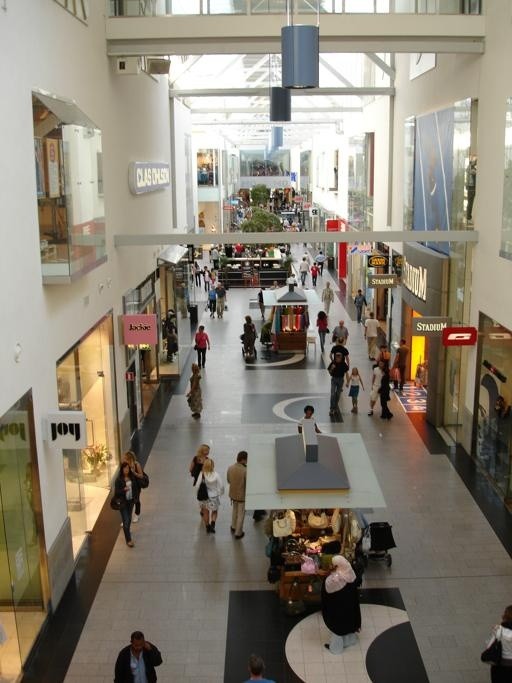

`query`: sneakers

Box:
[132,512,140,523]
[368,410,374,416]
[191,412,201,420]
[230,525,235,533]
[328,409,335,417]
[120,522,124,527]
[235,532,245,540]
[205,520,216,534]
[126,541,134,548]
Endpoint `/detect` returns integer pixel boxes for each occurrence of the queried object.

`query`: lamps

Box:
[270,53,291,121]
[282,0,319,89]
[272,127,283,146]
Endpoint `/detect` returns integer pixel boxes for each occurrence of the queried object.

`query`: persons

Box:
[243,314,257,355]
[111,462,137,547]
[330,336,350,366]
[251,510,266,522]
[194,325,210,367]
[368,361,382,416]
[120,451,150,526]
[364,311,384,360]
[321,281,334,315]
[189,444,210,516]
[284,217,308,246]
[242,654,277,683]
[186,362,203,419]
[287,273,297,287]
[378,367,393,420]
[392,338,409,391]
[331,320,348,346]
[315,311,329,353]
[270,188,291,215]
[298,405,321,434]
[257,287,267,320]
[322,555,362,655]
[194,243,228,319]
[347,367,365,411]
[480,604,511,682]
[299,250,327,286]
[114,631,162,681]
[226,450,248,539]
[194,459,224,533]
[377,345,391,369]
[354,289,367,323]
[327,352,349,417]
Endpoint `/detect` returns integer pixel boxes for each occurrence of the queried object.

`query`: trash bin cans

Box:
[328,257,334,269]
[188,305,197,322]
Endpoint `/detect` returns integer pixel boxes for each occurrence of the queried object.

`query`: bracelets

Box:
[364,333,366,336]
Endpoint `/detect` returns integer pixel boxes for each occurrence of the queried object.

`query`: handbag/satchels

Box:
[193,344,198,351]
[197,471,210,502]
[480,625,503,668]
[388,363,402,383]
[109,485,129,511]
[326,327,329,334]
[265,509,365,618]
[134,462,149,489]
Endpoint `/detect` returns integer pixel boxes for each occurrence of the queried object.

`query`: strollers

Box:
[358,519,395,568]
[240,327,258,358]
